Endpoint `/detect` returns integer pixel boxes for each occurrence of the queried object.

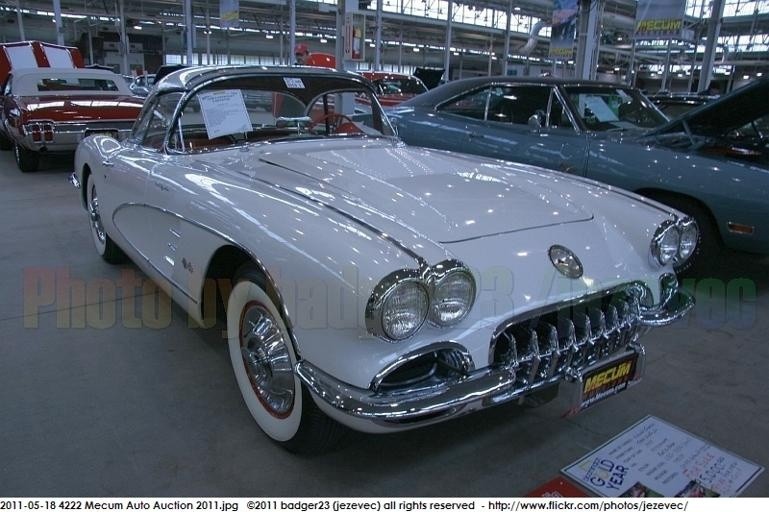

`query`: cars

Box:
[0,39,167,172]
[347,68,768,278]
[72,58,702,450]
[131,51,482,133]
